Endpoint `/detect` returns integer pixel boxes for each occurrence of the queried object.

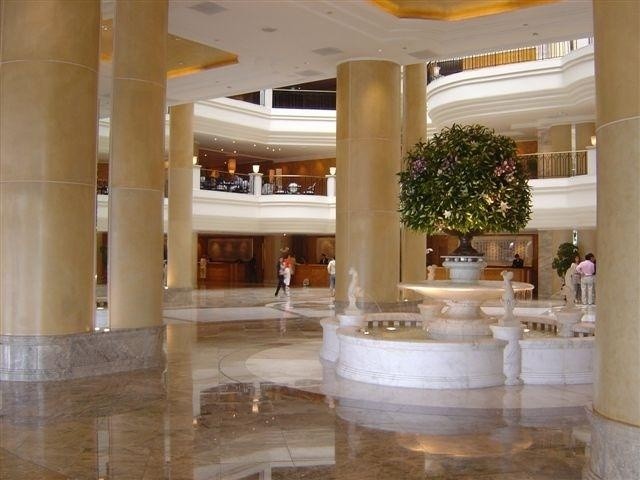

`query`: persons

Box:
[200,172,316,195]
[564,254,582,305]
[576,255,595,305]
[588,254,596,305]
[199,252,338,297]
[512,254,523,267]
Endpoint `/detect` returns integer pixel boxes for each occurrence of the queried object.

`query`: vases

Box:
[444,228,483,258]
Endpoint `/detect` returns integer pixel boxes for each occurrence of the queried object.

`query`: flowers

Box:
[398,122,534,234]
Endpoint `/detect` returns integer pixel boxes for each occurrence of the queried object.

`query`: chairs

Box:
[196,172,319,194]
